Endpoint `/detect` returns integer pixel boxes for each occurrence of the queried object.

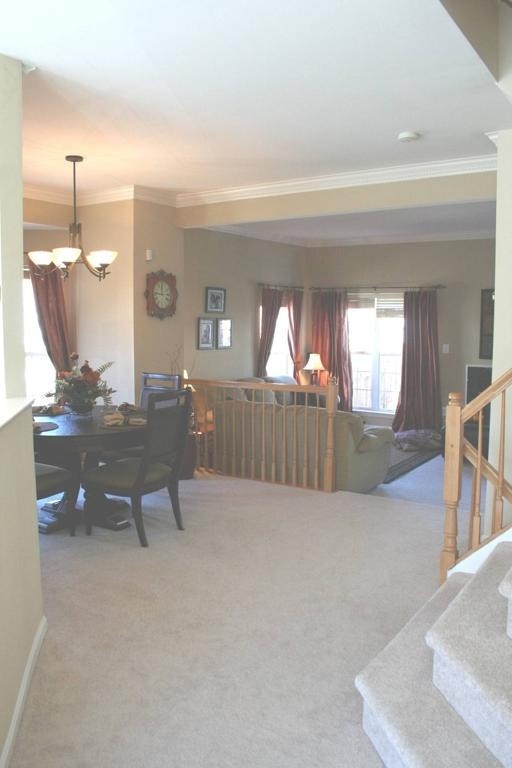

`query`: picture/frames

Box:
[197,317,216,350]
[204,286,226,313]
[215,317,233,349]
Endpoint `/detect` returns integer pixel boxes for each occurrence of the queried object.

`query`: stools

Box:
[34,456,78,539]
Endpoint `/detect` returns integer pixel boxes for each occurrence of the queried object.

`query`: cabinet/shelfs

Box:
[439,421,490,466]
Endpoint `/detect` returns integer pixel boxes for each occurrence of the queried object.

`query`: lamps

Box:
[303,353,325,384]
[28,155,118,281]
[183,367,197,432]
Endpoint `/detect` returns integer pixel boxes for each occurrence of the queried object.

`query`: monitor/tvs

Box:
[465,364,492,419]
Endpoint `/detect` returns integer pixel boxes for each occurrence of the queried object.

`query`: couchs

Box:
[200,375,393,494]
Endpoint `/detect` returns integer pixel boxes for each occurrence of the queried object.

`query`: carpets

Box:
[384,429,442,483]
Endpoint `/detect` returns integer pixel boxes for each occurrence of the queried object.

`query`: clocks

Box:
[144,267,177,320]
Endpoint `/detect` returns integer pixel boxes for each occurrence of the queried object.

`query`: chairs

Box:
[140,371,179,413]
[80,387,191,547]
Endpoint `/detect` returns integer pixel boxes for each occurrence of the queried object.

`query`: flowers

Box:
[44,352,117,414]
